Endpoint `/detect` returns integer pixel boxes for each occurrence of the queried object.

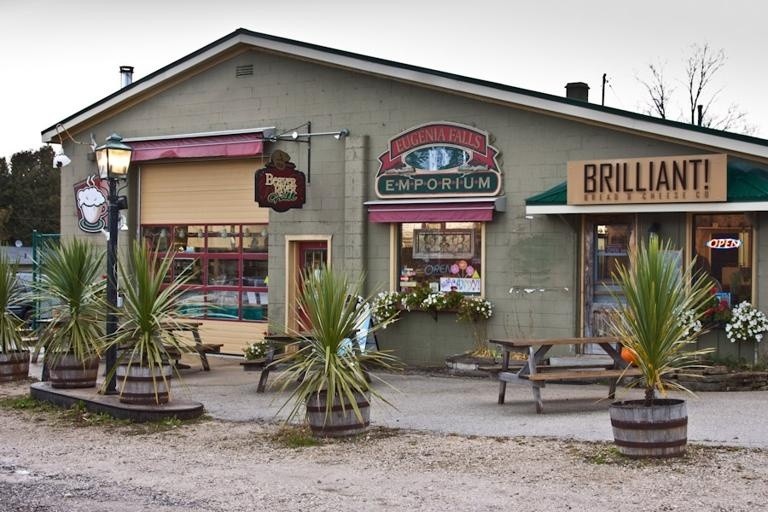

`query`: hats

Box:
[4,270,65,331]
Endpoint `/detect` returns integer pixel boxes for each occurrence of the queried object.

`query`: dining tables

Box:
[0,238,30,384]
[260,260,409,439]
[84,230,216,405]
[19,232,112,388]
[597,232,719,459]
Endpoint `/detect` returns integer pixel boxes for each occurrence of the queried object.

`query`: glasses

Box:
[51,123,100,169]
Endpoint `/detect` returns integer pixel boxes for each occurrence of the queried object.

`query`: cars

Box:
[78,199,109,224]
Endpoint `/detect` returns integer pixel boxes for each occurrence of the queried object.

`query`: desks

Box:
[94,130,138,398]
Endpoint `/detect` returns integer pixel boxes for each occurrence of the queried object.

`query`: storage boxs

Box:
[240,351,295,368]
[476,365,609,376]
[161,343,225,360]
[516,368,654,388]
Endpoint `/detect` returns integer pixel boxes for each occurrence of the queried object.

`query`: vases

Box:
[369,286,495,326]
[676,287,768,344]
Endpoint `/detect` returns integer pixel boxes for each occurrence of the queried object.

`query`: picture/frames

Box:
[155,321,209,371]
[488,336,636,414]
[256,329,373,392]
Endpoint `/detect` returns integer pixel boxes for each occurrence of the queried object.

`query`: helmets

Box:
[77,217,107,232]
